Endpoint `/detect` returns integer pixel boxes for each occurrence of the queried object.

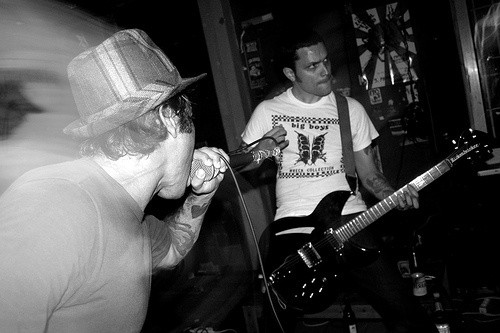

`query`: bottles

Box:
[433,293,451,333]
[343,296,357,333]
[410,250,429,300]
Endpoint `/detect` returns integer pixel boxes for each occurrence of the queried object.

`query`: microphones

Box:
[190,149,280,181]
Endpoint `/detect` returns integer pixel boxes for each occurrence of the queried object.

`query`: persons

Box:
[231,30,440,333]
[0,28,230,333]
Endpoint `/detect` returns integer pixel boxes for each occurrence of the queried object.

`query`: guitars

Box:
[257,126,492,312]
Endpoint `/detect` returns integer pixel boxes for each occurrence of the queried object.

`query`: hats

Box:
[63,28,207,137]
